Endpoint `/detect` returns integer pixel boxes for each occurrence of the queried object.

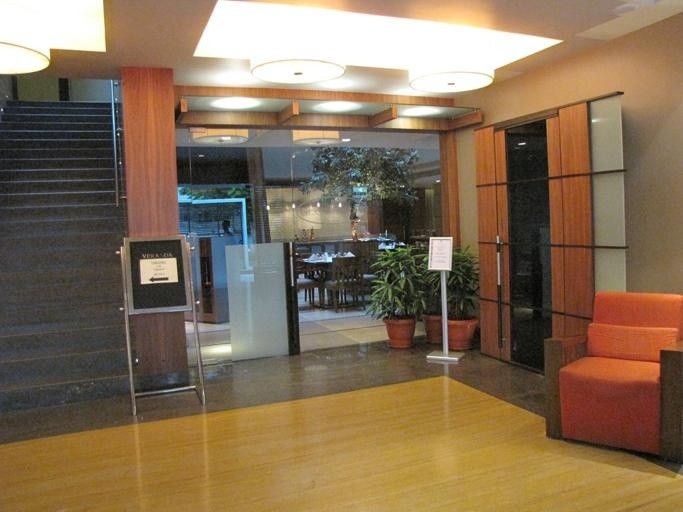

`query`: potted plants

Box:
[416,251,450,344]
[447,244,480,351]
[367,244,423,348]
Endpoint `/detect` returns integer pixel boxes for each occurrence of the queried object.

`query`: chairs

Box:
[544,292,683,465]
[295,251,379,312]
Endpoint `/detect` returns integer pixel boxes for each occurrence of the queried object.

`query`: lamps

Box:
[293,129,340,145]
[192,128,249,144]
[0,33,51,75]
[250,48,345,85]
[408,58,494,93]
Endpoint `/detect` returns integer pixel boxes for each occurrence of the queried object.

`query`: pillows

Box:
[587,322,679,363]
